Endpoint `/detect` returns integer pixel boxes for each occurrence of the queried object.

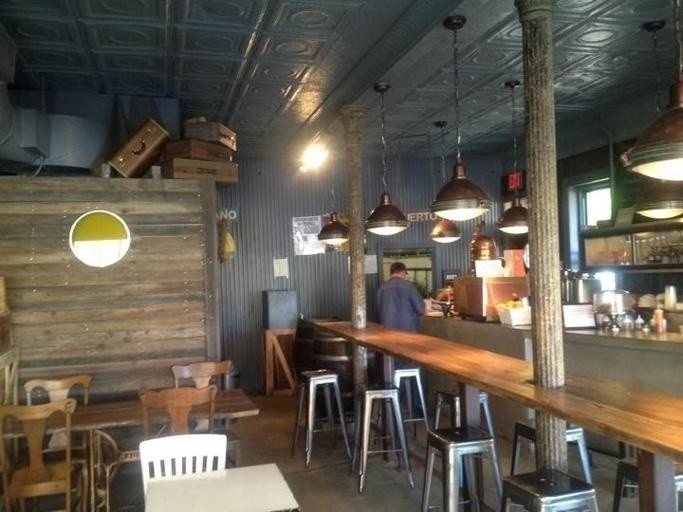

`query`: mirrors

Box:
[69,209,131,268]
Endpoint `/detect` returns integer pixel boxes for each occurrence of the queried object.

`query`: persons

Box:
[373,261,430,415]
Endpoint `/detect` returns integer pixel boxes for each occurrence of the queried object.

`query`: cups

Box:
[422,298,430,313]
[637,284,682,312]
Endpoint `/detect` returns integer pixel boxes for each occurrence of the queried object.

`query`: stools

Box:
[498,469,599,510]
[420,424,507,509]
[431,379,496,441]
[292,368,353,468]
[348,383,416,494]
[509,418,593,490]
[609,457,682,511]
[372,363,431,445]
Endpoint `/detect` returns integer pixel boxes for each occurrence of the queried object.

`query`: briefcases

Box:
[186,121,237,152]
[108,117,170,179]
[166,140,239,188]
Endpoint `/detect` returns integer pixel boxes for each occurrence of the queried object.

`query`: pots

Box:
[559,265,600,304]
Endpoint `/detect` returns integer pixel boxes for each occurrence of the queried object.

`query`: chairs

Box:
[0,346,240,512]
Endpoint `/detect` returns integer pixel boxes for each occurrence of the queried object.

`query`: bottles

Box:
[618,234,682,265]
[511,297,521,309]
[604,306,668,339]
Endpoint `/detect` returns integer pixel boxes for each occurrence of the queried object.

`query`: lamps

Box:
[620,2,682,182]
[634,180,682,222]
[365,81,409,237]
[427,121,461,244]
[495,78,531,236]
[318,154,350,245]
[428,15,492,224]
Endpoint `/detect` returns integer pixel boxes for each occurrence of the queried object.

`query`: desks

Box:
[145,462,300,512]
